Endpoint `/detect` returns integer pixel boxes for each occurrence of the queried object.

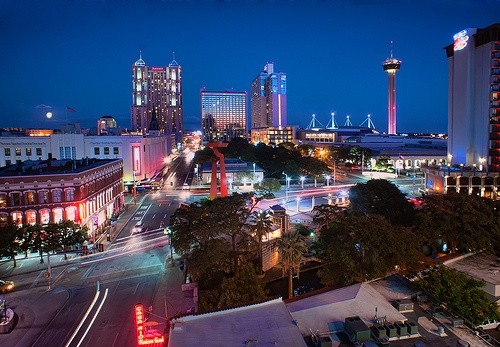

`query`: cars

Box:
[474,318,499,331]
[0,280,15,293]
[183,183,189,190]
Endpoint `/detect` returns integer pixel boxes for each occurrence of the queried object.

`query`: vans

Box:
[133,221,145,233]
